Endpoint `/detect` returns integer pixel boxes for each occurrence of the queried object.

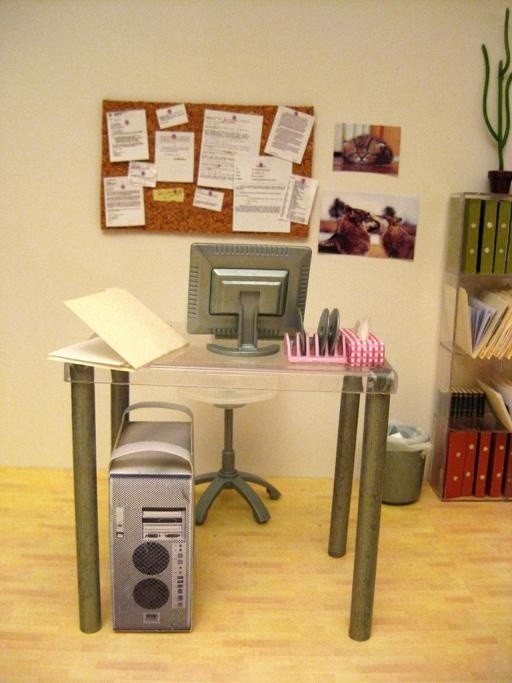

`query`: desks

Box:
[47,336,399,641]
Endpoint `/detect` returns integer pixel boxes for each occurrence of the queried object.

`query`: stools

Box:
[176,390,280,523]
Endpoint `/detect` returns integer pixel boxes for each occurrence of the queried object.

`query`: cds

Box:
[296,308,306,355]
[317,308,330,354]
[328,308,340,355]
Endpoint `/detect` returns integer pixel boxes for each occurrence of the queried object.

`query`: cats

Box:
[339,135,394,167]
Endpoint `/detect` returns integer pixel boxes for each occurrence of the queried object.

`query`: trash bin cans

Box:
[381,423,429,505]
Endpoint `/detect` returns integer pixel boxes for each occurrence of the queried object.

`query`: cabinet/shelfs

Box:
[427,194,512,501]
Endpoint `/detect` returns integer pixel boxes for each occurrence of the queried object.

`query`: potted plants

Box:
[482,7,512,194]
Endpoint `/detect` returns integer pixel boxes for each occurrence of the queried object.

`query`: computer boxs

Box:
[108,400,195,633]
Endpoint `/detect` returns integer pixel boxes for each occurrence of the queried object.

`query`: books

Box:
[437,288,512,431]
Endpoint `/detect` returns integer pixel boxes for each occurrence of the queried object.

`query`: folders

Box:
[445,191,512,275]
[429,416,512,499]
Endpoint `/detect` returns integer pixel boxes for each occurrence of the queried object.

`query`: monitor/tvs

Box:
[187,243,312,357]
